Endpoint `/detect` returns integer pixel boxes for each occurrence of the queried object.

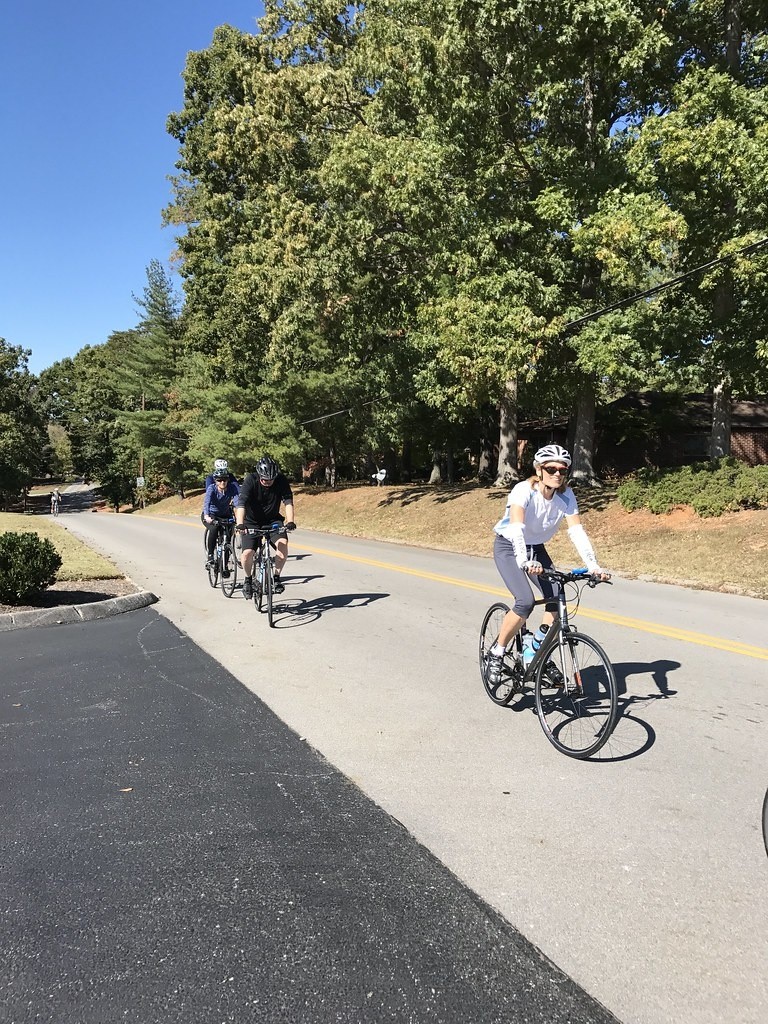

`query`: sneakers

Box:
[208,554,214,564]
[543,660,569,685]
[486,650,504,686]
[274,582,285,594]
[242,579,253,599]
[223,567,229,579]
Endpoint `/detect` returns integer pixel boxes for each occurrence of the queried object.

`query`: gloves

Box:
[235,524,248,530]
[285,522,296,531]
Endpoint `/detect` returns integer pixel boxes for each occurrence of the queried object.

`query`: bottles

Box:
[214,547,217,560]
[217,545,222,558]
[531,624,549,656]
[523,629,534,668]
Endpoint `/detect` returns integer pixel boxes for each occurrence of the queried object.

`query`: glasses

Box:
[216,479,228,482]
[540,466,571,476]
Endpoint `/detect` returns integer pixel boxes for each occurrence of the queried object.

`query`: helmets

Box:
[255,458,279,481]
[533,444,572,468]
[212,467,230,478]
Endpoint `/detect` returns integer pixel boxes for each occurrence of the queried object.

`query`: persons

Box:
[200,459,242,577]
[51,488,62,513]
[236,458,297,600]
[486,444,612,687]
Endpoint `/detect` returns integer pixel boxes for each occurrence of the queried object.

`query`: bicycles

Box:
[204,505,243,569]
[479,565,619,760]
[203,519,237,598]
[235,524,292,627]
[53,499,60,517]
